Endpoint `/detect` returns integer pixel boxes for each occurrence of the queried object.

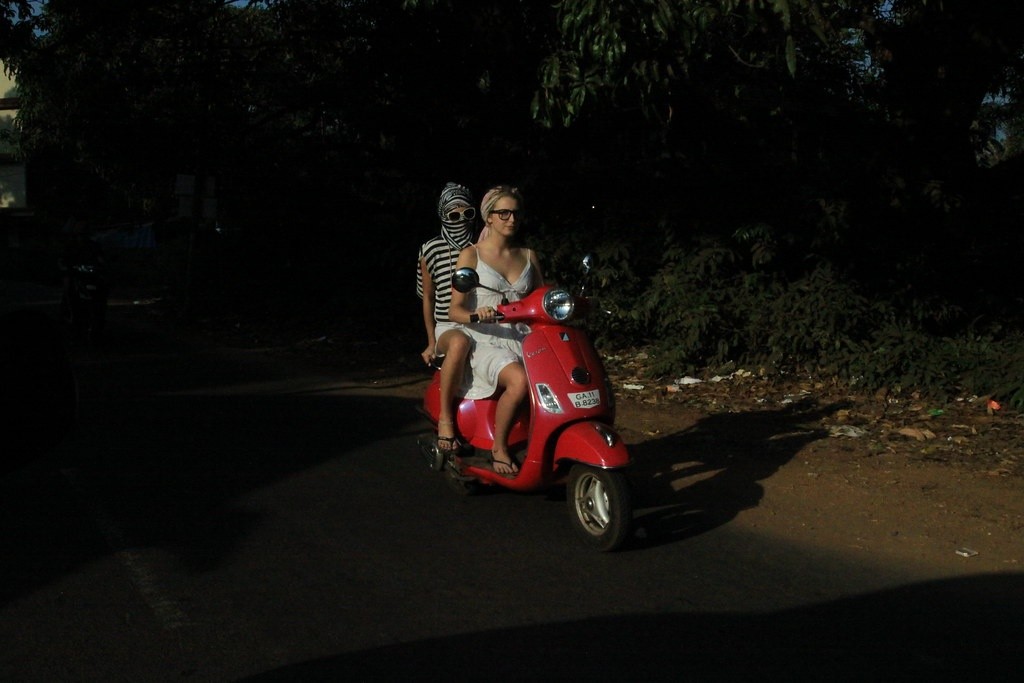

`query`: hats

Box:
[438,182,473,220]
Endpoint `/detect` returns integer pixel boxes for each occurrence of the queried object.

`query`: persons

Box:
[450,186,600,475]
[417,182,475,451]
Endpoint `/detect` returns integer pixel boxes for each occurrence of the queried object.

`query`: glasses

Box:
[445,206,475,223]
[489,209,525,220]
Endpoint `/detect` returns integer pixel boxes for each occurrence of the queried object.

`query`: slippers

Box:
[490,451,520,476]
[435,427,461,453]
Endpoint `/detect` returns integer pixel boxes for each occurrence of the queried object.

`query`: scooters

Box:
[410,251,631,554]
[55,262,113,355]
